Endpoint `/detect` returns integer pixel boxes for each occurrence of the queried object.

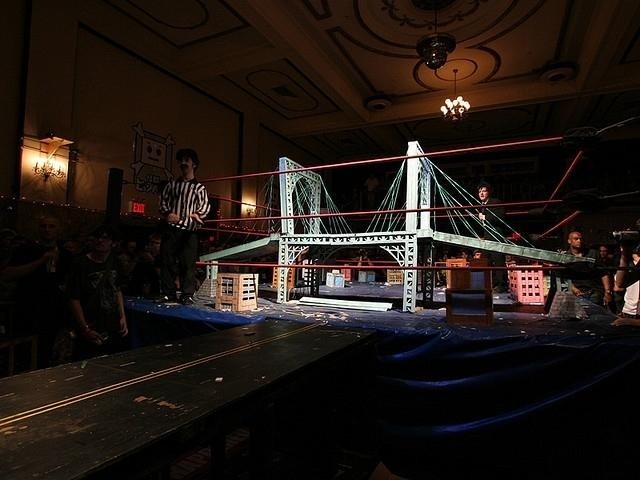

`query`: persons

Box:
[1,218,286,364]
[564,230,614,306]
[438,248,519,288]
[157,148,210,304]
[364,173,379,207]
[598,220,640,326]
[474,182,508,293]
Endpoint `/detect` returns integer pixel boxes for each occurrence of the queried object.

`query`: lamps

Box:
[415,1,456,69]
